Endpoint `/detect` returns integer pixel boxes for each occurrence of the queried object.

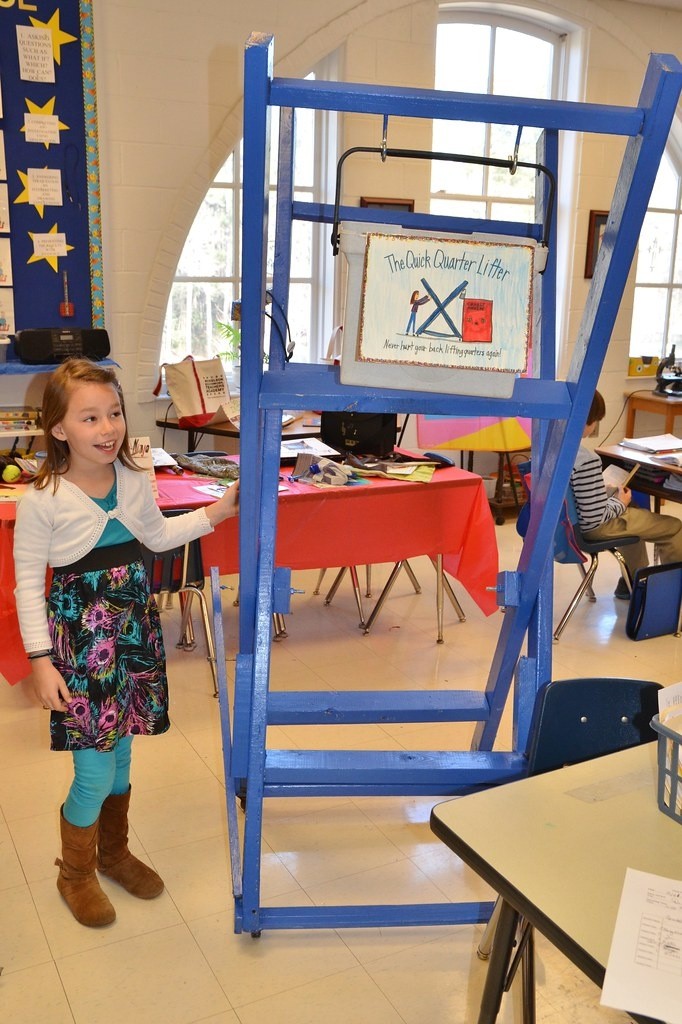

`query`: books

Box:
[599,462,641,499]
[618,434,682,497]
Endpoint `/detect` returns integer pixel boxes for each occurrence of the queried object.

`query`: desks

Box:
[0,423,44,455]
[594,444,682,566]
[0,446,499,645]
[155,409,404,454]
[623,389,682,506]
[430,740,682,1024]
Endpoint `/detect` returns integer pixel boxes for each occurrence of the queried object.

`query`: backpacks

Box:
[320,412,398,456]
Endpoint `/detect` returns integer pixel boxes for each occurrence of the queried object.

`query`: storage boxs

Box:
[629,357,659,376]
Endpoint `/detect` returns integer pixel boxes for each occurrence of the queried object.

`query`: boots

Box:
[54,802,116,927]
[96,783,164,900]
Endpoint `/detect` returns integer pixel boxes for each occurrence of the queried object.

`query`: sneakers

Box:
[613,575,632,600]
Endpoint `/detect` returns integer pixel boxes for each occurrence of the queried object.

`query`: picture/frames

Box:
[360,196,414,213]
[584,209,609,279]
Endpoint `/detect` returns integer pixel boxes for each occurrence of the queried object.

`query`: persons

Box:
[11,360,241,927]
[569,387,682,600]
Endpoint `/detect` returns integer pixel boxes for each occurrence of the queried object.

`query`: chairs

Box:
[517,457,640,646]
[477,678,665,960]
[166,450,287,643]
[137,509,219,699]
[324,453,464,634]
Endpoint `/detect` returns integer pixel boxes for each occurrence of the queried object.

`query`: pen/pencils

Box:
[655,449,678,454]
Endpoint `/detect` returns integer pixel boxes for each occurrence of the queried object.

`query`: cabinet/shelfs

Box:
[467,448,532,525]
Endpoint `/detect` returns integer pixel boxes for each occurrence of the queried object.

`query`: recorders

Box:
[14,327,111,366]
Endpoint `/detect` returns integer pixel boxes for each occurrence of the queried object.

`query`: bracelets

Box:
[26,651,52,662]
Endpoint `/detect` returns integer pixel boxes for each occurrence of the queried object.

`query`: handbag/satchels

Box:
[153,355,231,430]
